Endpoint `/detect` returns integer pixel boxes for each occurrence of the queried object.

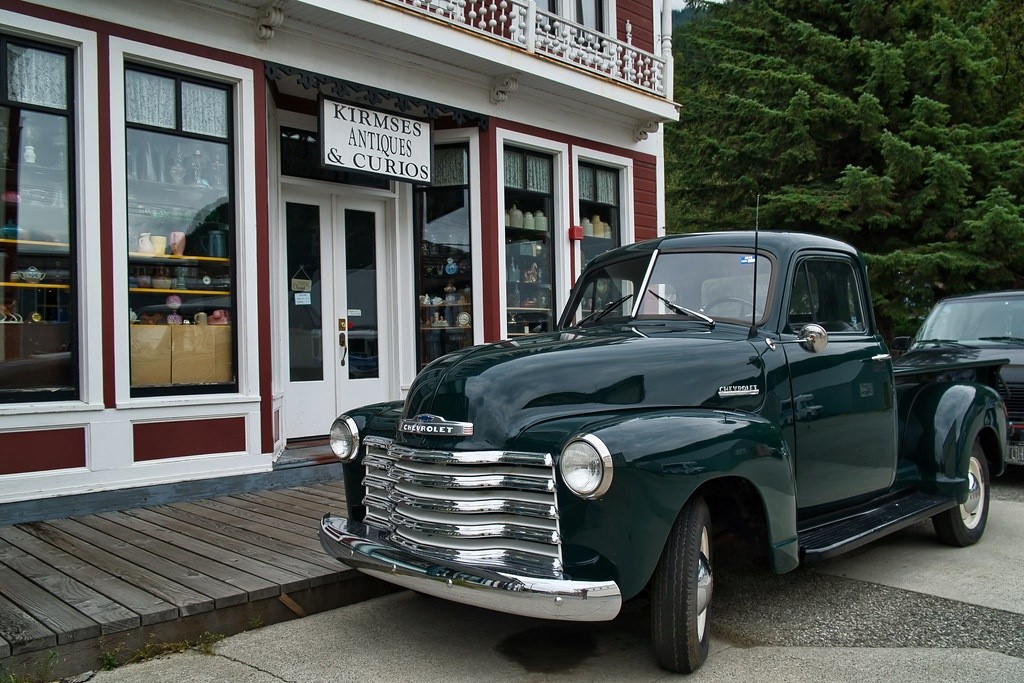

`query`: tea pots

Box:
[18,265,46,284]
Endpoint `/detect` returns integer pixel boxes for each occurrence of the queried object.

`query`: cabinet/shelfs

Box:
[421,226,475,365]
[580,234,620,321]
[127,178,234,385]
[0,158,78,386]
[505,225,557,339]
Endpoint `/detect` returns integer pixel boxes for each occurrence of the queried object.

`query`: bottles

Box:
[505,204,611,239]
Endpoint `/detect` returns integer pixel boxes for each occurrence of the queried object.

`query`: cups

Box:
[128,192,231,327]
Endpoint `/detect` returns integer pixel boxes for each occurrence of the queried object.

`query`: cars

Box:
[313,194,1007,666]
[890,291,1023,477]
